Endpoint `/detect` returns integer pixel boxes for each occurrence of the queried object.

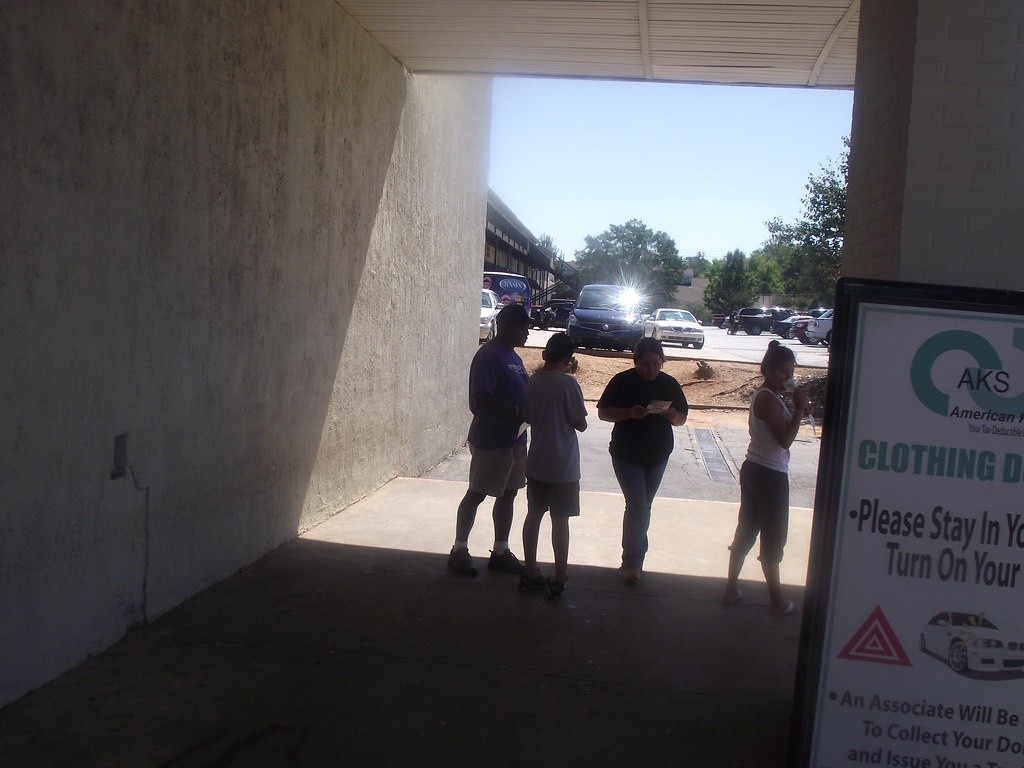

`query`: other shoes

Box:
[623,564,642,583]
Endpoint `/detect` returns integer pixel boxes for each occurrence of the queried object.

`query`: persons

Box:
[595,336,691,586]
[722,340,810,616]
[444,299,533,582]
[519,331,588,598]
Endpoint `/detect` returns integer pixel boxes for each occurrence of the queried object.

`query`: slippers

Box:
[766,600,801,616]
[721,591,750,608]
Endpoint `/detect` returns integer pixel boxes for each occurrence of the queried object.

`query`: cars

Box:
[565,282,649,354]
[712,292,835,349]
[642,305,706,350]
[480,272,575,343]
[918,609,1024,676]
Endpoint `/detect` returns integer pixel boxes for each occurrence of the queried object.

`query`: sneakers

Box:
[545,575,566,599]
[518,571,548,590]
[447,546,479,576]
[488,549,526,571]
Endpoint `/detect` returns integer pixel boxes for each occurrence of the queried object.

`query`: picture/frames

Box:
[786,272,1024,768]
